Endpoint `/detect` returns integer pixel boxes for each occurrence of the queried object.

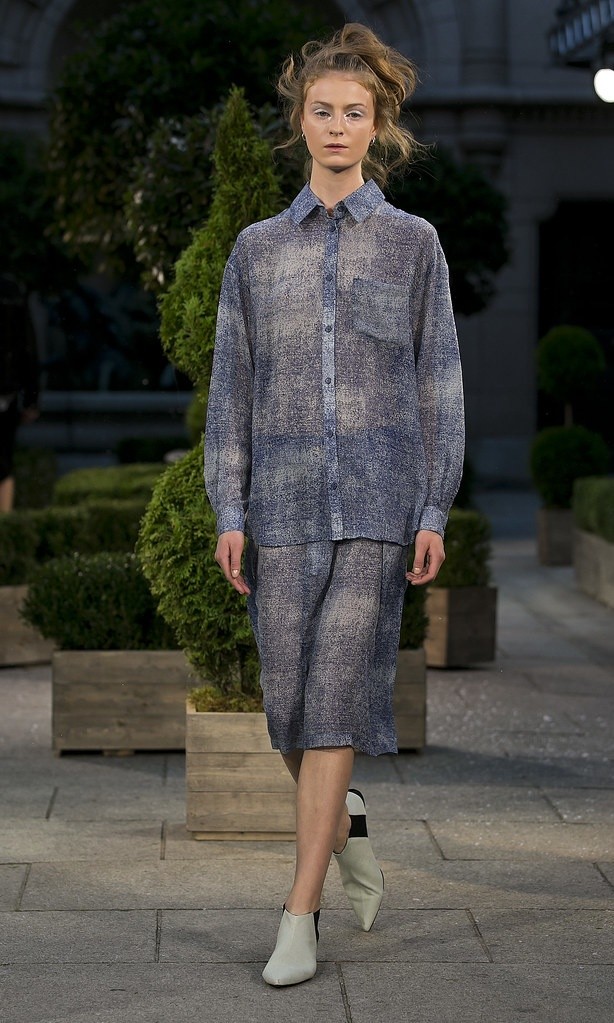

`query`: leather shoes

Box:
[332,788,384,932]
[262,904,320,987]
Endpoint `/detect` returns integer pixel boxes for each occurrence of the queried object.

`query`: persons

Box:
[204,24,465,987]
